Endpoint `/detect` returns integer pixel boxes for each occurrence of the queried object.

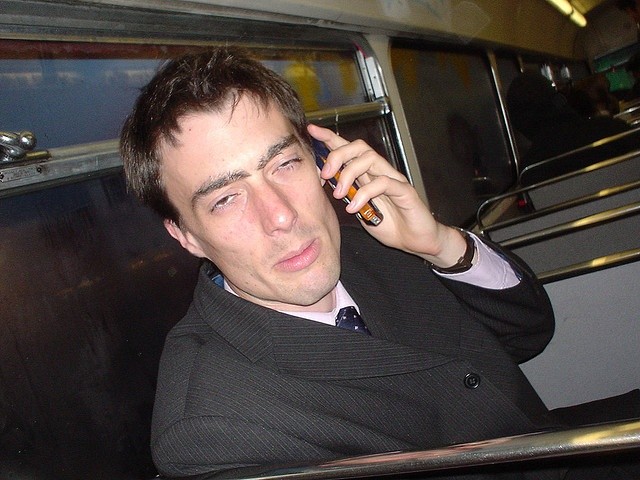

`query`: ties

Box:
[335,306,373,336]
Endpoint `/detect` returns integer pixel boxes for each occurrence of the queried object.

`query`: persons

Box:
[119,44,571,479]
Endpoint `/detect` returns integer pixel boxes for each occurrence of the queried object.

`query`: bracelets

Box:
[428,225,476,275]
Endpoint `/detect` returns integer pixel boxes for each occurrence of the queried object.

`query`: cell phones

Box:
[315,152,384,227]
